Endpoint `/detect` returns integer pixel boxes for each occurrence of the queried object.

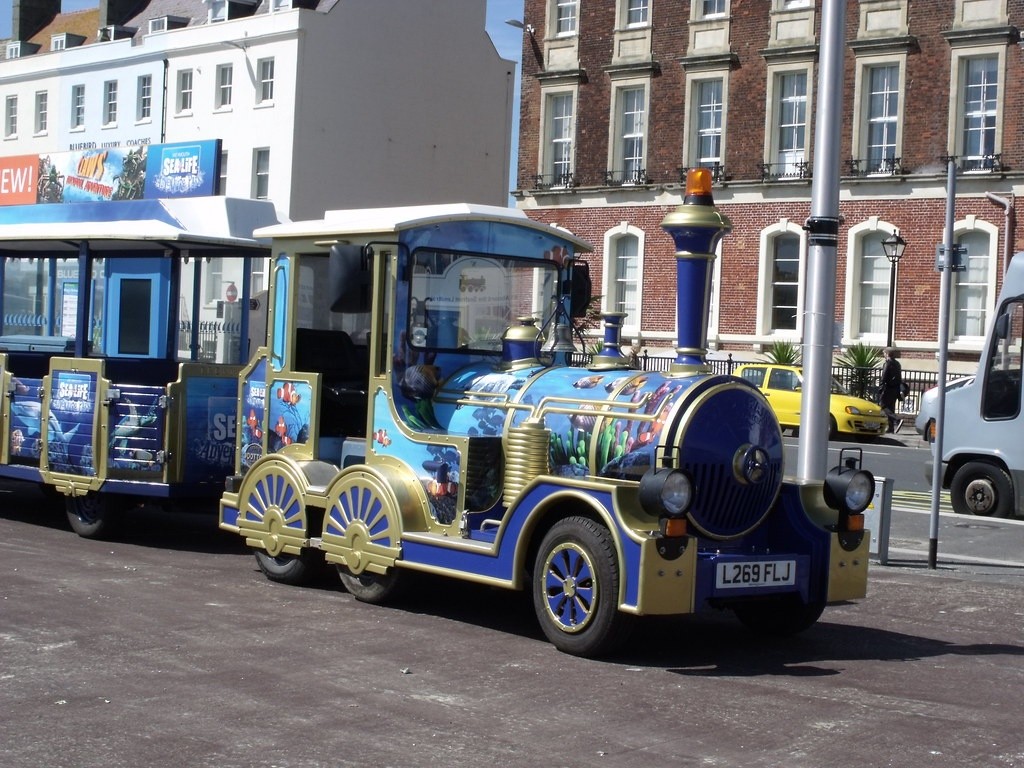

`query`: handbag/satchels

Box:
[897,383,910,401]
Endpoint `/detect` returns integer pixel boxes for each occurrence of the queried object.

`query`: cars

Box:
[915,374,975,441]
[734,362,888,442]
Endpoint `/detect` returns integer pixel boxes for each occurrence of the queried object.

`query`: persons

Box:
[879,346,904,434]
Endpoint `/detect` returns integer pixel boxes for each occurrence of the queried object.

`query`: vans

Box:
[926,253,1024,521]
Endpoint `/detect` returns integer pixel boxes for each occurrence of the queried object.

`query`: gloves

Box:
[877,390,882,394]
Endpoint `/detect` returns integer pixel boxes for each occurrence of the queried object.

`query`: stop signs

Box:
[227,285,237,302]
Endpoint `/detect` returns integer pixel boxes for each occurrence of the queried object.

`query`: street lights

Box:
[880,230,908,356]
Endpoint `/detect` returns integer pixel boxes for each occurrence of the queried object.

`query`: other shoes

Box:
[886,430,894,433]
[895,419,904,433]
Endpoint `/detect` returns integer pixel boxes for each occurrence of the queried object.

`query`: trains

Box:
[0,168,876,659]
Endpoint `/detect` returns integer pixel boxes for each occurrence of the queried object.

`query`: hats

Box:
[882,347,896,357]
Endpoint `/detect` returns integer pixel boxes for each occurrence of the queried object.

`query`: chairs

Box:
[296,327,368,435]
